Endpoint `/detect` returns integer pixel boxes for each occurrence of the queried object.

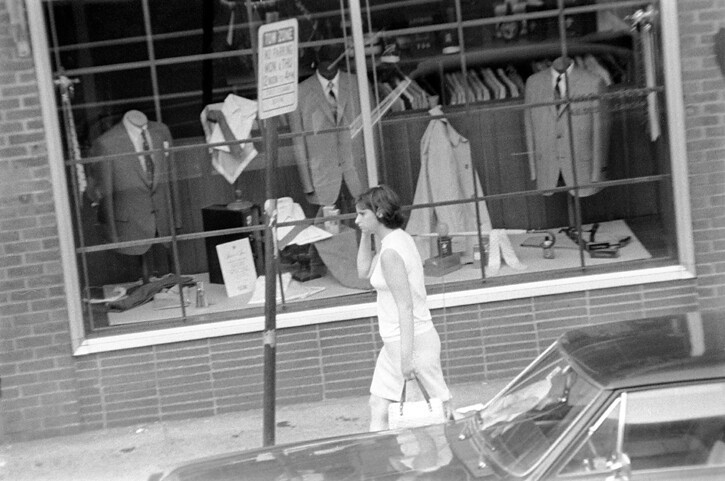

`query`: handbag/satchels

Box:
[389,375,443,428]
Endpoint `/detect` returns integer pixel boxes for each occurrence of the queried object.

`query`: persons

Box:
[92,110,183,256]
[287,59,379,207]
[354,184,455,433]
[523,56,611,197]
[387,424,453,481]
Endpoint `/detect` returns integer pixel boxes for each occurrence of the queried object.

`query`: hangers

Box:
[445,66,525,106]
[378,76,428,113]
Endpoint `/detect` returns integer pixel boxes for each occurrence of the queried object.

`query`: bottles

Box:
[196,281,207,308]
[543,236,553,259]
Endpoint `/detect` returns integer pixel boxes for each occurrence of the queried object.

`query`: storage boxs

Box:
[201,203,265,285]
[423,252,462,278]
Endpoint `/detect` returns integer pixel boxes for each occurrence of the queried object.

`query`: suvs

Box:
[146,307,725,481]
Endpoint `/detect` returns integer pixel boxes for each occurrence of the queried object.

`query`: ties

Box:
[554,76,562,112]
[327,83,337,119]
[141,130,153,183]
